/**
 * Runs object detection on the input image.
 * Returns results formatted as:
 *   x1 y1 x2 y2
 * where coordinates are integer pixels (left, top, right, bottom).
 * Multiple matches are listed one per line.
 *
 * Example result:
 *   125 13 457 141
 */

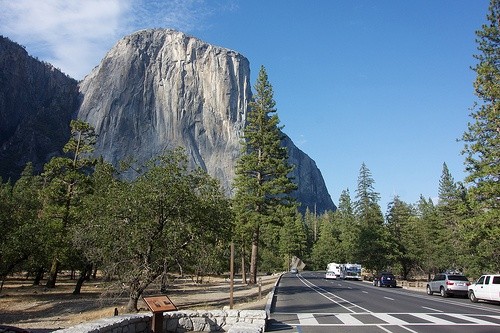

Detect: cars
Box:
323 271 337 280
372 272 397 288
290 267 298 274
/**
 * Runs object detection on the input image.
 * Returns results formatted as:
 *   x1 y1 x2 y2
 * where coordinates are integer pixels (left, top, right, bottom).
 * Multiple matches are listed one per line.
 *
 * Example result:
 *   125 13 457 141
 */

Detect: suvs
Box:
468 275 500 304
425 273 471 297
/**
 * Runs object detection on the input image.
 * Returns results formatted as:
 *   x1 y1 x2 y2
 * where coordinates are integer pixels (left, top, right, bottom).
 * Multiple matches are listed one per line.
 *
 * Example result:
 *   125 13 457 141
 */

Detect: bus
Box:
338 264 362 280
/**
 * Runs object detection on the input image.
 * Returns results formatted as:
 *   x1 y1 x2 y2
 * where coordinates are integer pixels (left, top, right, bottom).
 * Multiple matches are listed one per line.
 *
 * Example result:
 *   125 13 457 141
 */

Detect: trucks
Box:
326 262 340 276
